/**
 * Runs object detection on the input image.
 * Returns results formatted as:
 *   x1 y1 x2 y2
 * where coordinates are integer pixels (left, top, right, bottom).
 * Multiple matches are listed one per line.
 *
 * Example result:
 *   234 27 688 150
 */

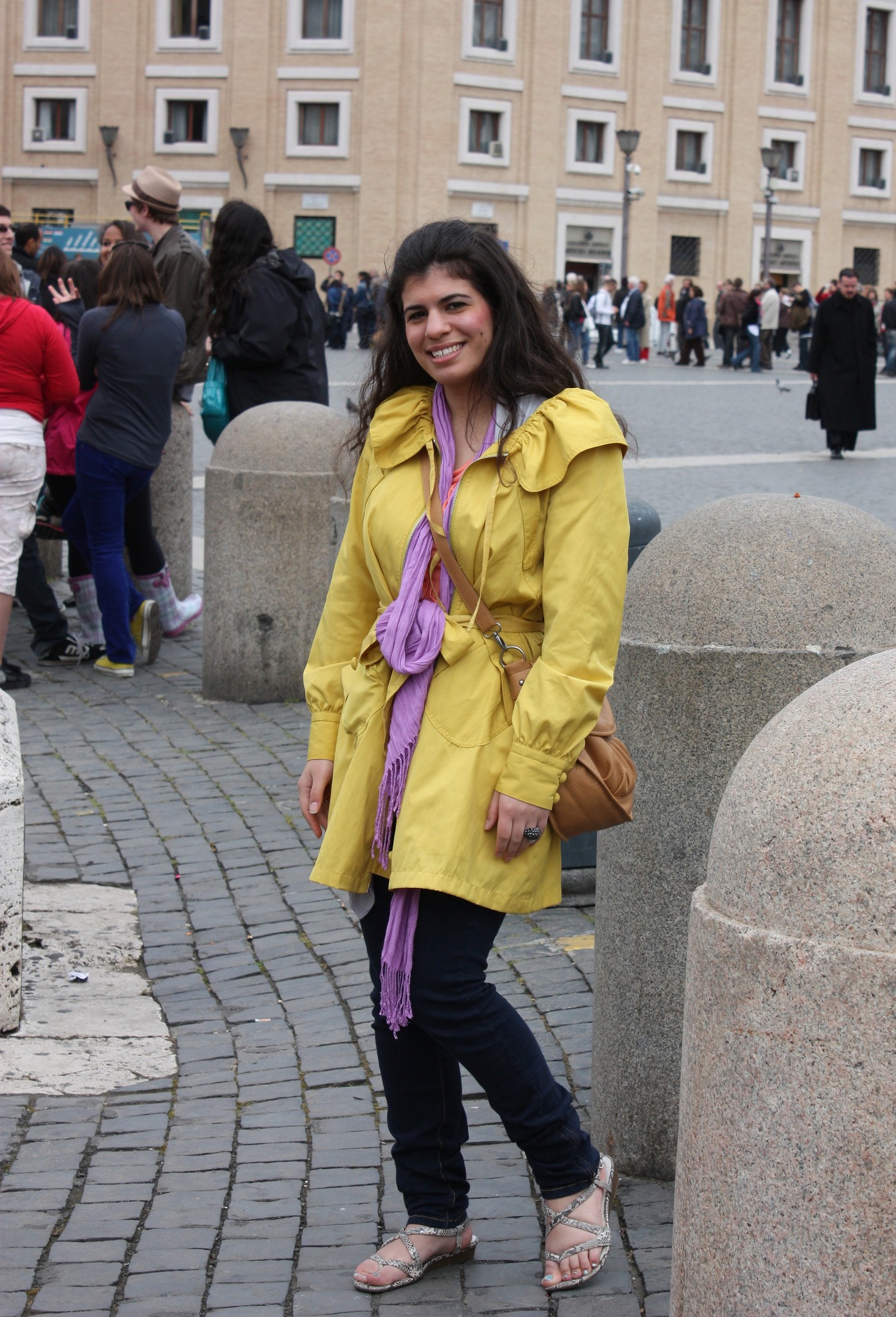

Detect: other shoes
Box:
830 449 844 459
658 350 704 366
613 347 647 365
594 357 603 368
785 349 792 360
62 597 75 607
581 363 594 368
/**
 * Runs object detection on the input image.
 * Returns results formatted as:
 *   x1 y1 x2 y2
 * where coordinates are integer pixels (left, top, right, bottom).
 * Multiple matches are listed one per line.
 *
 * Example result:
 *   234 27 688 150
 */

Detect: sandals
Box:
355 1213 478 1291
542 1151 615 1290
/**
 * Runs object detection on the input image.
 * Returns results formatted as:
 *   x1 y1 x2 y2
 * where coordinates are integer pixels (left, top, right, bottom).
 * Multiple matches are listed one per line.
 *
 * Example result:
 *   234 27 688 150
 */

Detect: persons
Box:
42 241 190 678
299 219 638 1293
0 163 212 664
317 265 896 381
198 200 330 448
0 242 80 689
804 268 877 460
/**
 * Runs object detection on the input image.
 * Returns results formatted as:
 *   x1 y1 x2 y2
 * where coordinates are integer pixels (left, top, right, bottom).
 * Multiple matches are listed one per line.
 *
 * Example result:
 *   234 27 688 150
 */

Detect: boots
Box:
66 573 107 656
135 562 204 636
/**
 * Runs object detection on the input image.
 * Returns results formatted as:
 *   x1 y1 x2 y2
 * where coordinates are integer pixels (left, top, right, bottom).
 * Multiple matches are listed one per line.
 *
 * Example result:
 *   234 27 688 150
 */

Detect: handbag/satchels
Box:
805 377 821 420
579 299 596 331
201 357 231 444
502 655 637 841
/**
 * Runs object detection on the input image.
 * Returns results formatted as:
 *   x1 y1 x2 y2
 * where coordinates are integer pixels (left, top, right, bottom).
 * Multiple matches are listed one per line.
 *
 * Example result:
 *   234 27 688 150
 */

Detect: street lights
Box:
759 146 785 281
614 129 642 278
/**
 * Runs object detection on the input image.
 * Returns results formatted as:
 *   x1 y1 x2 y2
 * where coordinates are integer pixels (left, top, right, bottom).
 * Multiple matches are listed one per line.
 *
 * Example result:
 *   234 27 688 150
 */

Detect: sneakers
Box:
94 655 134 677
130 599 160 663
37 632 94 662
0 655 32 690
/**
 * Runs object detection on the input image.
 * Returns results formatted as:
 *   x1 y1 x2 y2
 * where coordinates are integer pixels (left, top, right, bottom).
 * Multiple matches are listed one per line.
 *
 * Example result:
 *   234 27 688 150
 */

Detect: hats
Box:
122 166 183 213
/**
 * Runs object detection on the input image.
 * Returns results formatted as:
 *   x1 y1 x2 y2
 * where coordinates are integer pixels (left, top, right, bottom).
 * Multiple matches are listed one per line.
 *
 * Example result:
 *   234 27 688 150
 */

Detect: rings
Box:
525 827 541 840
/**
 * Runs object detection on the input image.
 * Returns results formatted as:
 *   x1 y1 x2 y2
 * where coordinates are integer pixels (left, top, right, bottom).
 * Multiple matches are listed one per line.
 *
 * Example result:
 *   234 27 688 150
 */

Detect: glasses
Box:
0 224 16 233
111 241 148 250
125 200 141 211
